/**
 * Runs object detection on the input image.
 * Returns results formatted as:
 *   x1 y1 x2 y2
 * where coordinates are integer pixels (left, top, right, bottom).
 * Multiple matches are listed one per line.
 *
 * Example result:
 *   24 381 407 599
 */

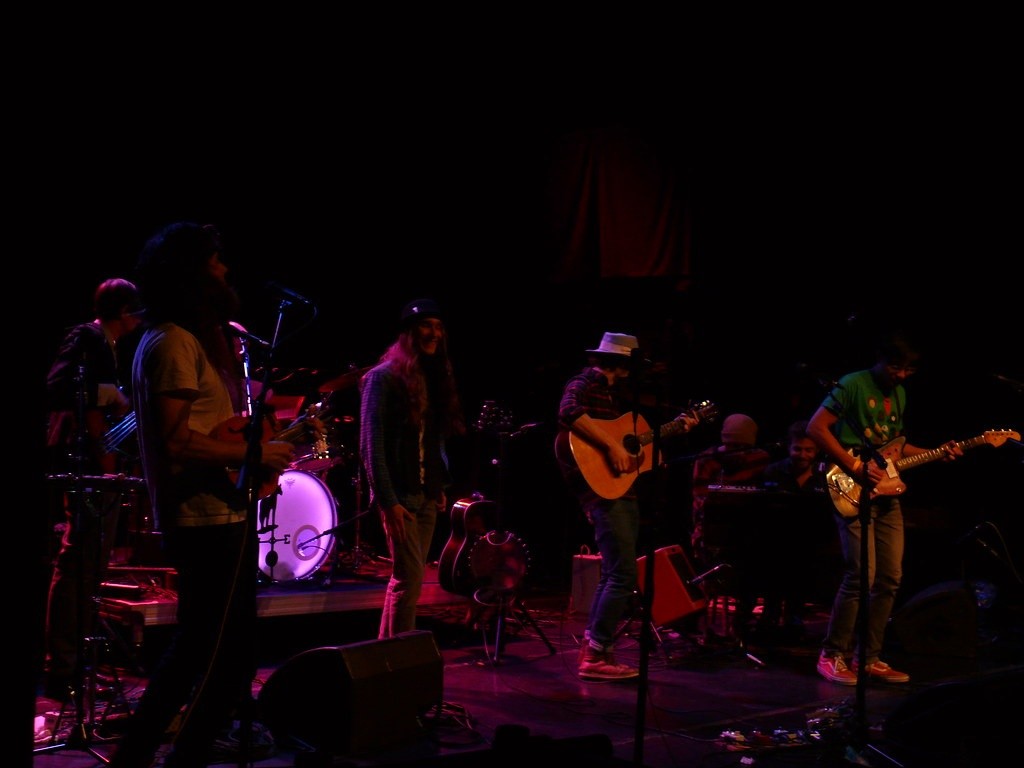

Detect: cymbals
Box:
317 366 374 393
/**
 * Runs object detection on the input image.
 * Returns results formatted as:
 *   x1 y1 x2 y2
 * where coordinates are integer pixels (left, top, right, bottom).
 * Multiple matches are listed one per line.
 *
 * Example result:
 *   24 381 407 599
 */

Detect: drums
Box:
286 419 343 472
125 455 163 536
256 468 339 581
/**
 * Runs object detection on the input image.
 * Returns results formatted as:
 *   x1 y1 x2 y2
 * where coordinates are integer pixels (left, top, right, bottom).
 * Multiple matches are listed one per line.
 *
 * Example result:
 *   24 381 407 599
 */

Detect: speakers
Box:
881 580 976 673
572 554 603 613
251 630 444 757
634 544 710 624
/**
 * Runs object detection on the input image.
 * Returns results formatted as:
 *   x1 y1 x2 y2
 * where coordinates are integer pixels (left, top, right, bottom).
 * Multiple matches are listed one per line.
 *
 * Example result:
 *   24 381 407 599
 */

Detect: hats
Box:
720 413 756 446
587 332 639 356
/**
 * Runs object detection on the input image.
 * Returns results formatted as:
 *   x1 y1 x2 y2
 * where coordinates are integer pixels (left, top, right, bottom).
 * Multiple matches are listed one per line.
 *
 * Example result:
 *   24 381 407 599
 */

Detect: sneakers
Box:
848 659 908 683
578 658 639 680
818 652 856 685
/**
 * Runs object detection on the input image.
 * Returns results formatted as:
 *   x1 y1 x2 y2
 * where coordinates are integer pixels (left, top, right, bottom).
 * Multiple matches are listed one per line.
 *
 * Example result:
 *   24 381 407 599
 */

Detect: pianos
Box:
692 479 826 503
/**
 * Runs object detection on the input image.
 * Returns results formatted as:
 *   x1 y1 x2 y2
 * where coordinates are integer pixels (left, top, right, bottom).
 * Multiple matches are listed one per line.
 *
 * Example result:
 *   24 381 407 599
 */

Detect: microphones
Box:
760 443 780 452
274 285 312 305
632 348 664 371
955 524 983 546
688 563 727 587
798 361 845 388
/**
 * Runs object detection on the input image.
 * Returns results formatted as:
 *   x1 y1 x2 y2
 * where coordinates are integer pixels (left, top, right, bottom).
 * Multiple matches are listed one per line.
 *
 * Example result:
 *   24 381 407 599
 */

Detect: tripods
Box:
31 491 132 765
333 453 383 576
456 431 559 663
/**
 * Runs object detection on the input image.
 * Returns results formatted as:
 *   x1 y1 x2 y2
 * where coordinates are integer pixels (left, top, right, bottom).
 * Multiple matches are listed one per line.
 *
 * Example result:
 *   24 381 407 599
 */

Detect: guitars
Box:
435 399 499 594
204 406 335 505
823 426 1022 520
83 400 138 475
554 398 719 506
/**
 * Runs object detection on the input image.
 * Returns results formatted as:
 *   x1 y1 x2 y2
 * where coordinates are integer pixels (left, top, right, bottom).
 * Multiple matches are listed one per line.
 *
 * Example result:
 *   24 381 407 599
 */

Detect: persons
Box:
554 332 699 679
358 300 467 639
44 278 146 697
132 222 328 768
806 327 963 686
691 414 836 655
